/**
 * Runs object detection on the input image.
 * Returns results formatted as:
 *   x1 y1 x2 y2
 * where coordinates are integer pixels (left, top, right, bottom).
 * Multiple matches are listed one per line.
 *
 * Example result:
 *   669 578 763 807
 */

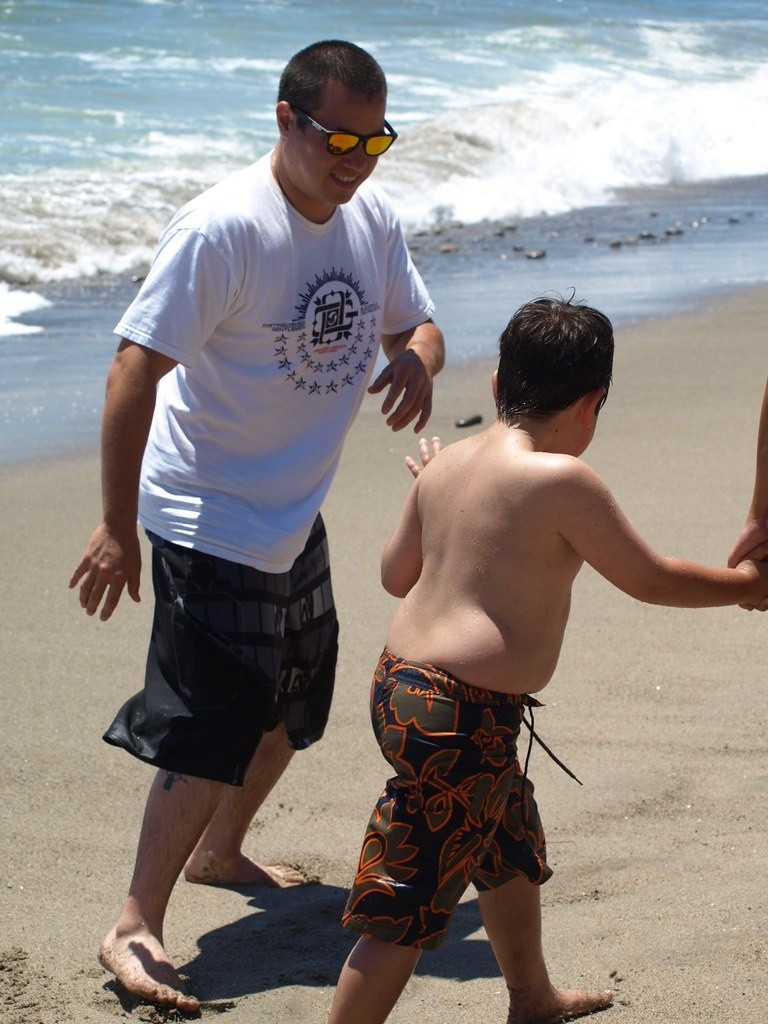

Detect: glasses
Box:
293 106 399 157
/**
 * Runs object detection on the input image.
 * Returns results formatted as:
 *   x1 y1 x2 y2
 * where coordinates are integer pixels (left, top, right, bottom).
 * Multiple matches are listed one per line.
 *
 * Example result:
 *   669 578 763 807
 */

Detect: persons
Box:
327 285 768 1024
70 40 445 1013
